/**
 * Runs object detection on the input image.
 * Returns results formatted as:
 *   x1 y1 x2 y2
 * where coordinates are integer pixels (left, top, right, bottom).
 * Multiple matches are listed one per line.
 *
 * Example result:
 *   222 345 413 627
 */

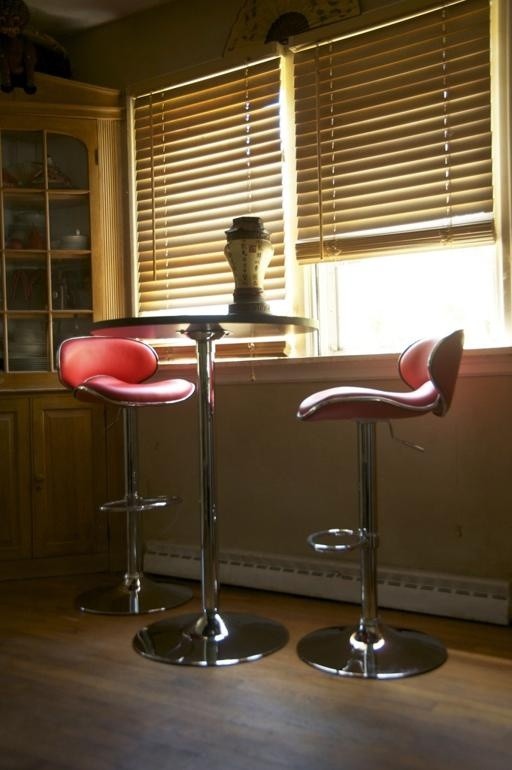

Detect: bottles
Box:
57 284 65 310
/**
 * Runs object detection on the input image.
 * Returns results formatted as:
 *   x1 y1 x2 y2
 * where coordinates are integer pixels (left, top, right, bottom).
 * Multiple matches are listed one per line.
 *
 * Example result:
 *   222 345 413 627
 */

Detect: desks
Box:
87 312 320 671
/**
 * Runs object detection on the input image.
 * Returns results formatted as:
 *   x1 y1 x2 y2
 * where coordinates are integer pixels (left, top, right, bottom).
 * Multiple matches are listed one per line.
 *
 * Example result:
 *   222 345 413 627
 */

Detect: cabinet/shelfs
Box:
1 388 126 582
1 68 129 389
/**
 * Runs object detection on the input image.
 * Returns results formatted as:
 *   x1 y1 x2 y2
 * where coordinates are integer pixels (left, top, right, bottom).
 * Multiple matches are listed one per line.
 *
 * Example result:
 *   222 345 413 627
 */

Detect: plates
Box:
0 355 50 372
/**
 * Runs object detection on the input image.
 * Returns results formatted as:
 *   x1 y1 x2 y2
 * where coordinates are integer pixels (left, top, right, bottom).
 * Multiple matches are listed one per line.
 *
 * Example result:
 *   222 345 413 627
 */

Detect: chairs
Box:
53 333 198 620
295 326 467 683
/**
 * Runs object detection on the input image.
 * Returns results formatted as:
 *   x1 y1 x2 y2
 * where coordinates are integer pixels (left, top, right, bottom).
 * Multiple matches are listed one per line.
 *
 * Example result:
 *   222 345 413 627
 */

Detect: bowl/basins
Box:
8 342 45 353
11 239 23 249
15 211 52 224
60 235 87 249
